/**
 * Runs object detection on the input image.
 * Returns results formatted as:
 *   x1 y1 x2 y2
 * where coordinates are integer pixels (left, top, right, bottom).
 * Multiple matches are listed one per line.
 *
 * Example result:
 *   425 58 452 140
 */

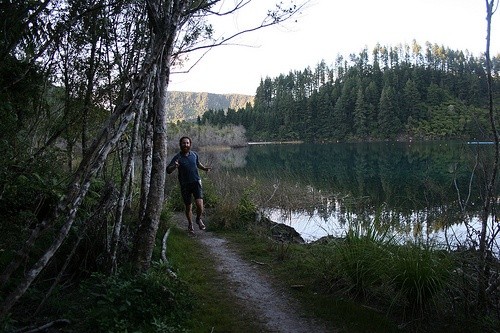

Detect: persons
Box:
166 137 212 234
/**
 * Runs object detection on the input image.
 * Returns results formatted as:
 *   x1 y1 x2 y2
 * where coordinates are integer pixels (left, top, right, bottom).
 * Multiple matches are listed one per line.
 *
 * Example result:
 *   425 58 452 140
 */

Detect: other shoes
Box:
188 222 194 233
196 217 205 230
